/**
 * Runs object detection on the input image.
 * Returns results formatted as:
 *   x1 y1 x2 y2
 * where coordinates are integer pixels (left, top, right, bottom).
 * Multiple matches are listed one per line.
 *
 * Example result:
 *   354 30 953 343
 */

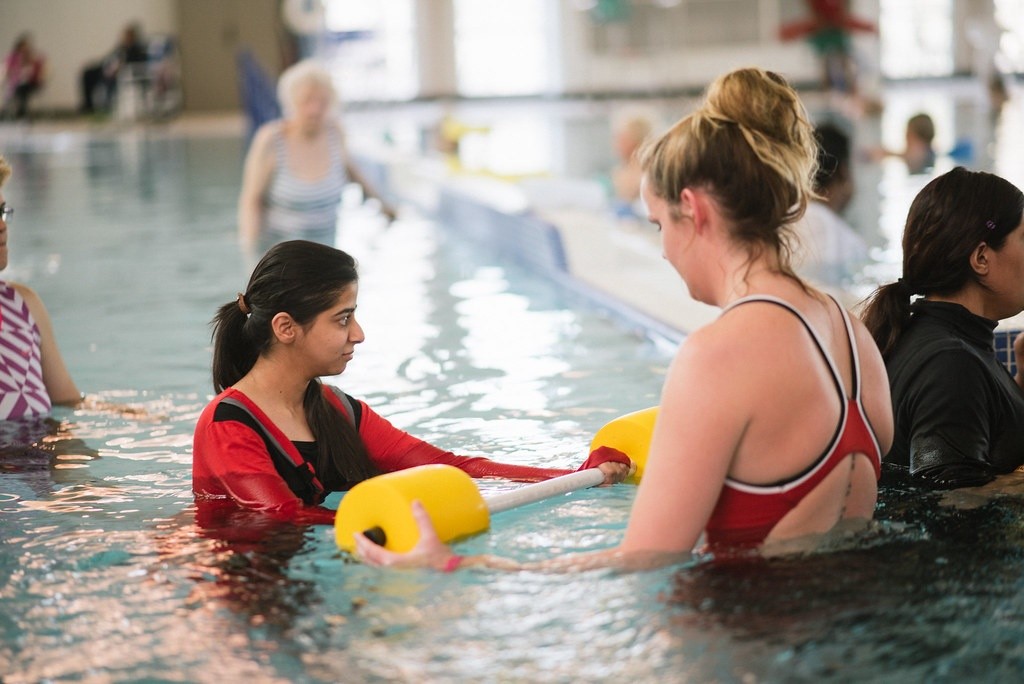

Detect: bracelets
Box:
444 557 463 571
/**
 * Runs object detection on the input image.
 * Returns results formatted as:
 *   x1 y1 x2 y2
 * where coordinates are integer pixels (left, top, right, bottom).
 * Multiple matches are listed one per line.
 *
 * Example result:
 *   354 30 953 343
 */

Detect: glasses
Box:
0 207 15 224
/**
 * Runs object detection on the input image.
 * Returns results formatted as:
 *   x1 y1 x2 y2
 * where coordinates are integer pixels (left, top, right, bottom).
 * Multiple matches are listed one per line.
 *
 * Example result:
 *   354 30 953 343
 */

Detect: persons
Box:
241 57 396 220
193 240 631 515
777 123 862 259
73 25 149 113
0 33 44 119
861 166 1024 473
0 158 81 422
907 114 935 149
353 69 894 565
